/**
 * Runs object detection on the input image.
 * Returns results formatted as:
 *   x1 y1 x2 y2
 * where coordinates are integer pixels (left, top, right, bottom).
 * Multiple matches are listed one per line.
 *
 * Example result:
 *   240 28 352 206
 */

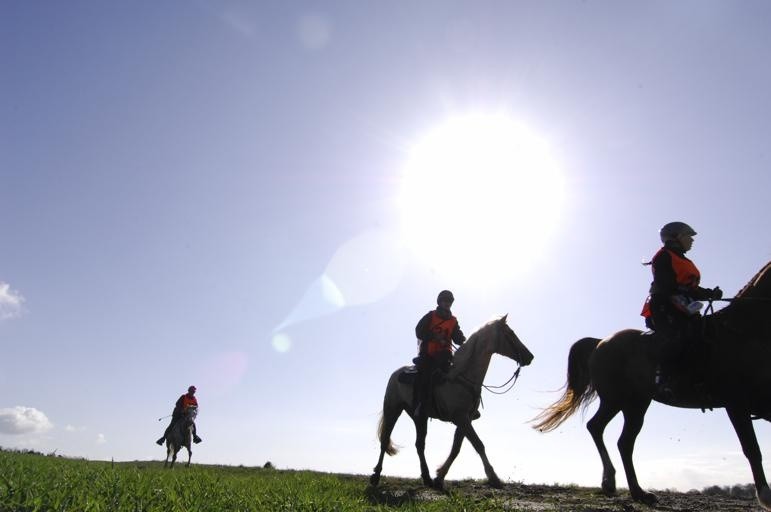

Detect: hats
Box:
189 386 196 392
437 290 454 304
660 221 696 241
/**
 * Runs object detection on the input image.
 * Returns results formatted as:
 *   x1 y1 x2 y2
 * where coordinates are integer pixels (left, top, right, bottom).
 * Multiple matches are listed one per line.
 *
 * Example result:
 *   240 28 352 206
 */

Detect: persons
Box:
156 385 202 445
413 287 482 420
640 220 725 405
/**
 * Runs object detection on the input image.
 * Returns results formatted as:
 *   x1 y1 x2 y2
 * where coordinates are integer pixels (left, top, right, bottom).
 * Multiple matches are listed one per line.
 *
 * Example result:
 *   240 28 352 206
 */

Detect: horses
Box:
164 403 199 470
533 261 770 512
368 310 533 492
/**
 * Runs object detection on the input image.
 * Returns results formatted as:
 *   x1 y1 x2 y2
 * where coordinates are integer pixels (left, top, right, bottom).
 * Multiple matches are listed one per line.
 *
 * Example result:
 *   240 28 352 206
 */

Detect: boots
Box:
193 436 200 443
158 437 165 444
